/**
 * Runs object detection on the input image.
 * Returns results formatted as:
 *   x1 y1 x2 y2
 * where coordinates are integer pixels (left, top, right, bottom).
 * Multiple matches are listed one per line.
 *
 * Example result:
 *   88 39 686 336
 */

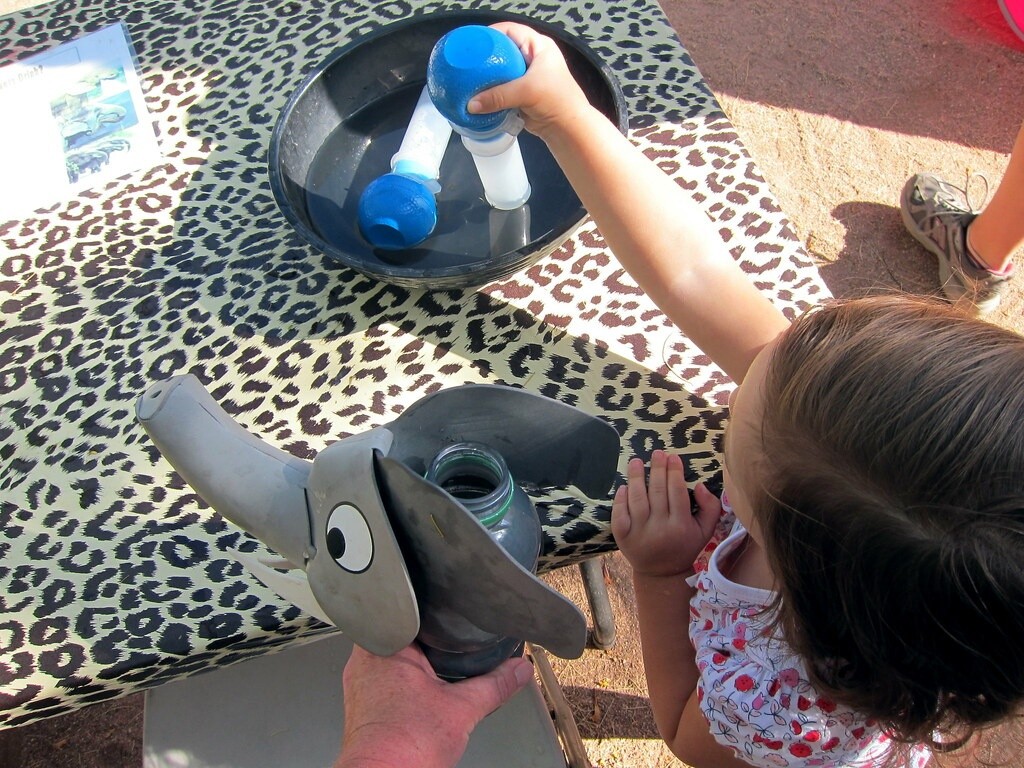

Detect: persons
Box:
900 118 1023 316
480 23 1024 767
331 639 533 768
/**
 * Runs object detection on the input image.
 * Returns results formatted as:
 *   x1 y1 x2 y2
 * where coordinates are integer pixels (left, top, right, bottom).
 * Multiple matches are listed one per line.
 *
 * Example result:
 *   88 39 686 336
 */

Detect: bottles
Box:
402 442 544 717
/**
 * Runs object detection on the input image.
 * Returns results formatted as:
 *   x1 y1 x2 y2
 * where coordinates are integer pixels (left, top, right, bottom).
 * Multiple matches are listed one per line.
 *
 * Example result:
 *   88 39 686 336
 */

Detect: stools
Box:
142 632 594 768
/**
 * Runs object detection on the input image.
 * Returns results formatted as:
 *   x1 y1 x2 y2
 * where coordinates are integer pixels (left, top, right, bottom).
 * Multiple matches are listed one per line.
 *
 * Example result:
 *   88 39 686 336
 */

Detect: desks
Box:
0 0 836 735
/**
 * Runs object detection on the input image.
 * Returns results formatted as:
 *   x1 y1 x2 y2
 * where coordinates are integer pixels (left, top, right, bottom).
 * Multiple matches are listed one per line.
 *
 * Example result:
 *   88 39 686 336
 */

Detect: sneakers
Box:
900 168 1015 316
